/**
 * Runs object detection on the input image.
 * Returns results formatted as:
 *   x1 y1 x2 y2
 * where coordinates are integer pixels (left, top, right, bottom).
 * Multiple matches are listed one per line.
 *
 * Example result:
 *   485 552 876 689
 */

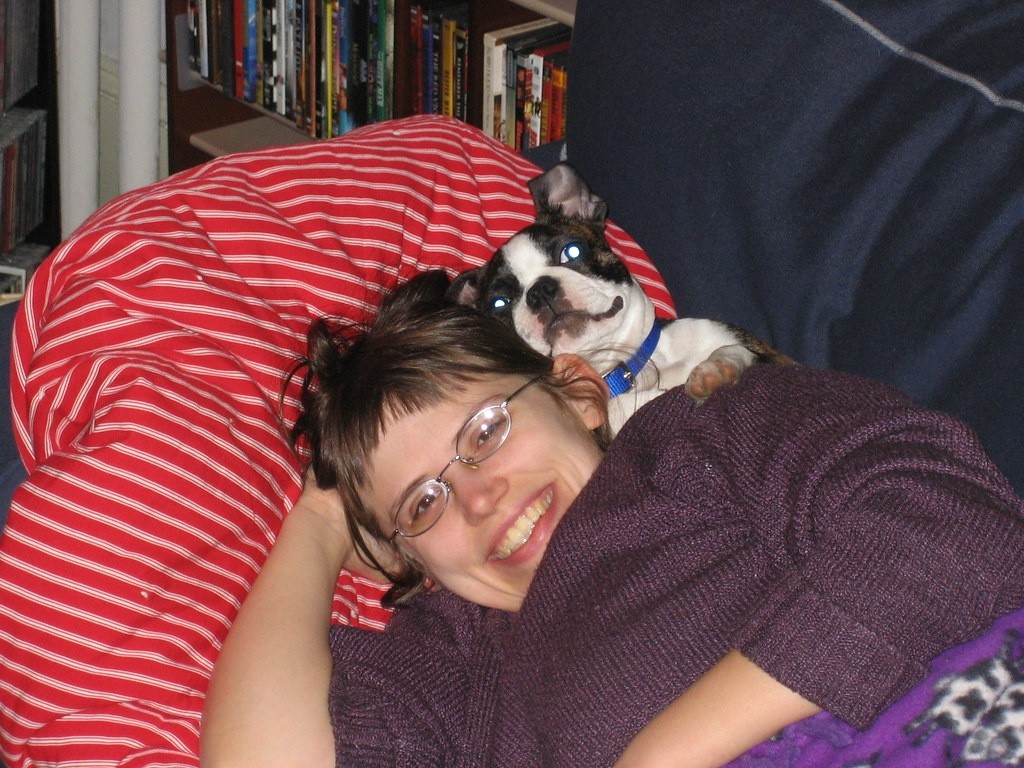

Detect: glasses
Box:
388 371 545 543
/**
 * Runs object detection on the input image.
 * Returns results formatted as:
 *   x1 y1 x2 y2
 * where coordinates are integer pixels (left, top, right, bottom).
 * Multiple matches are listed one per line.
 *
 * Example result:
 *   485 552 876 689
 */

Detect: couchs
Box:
0 0 1024 768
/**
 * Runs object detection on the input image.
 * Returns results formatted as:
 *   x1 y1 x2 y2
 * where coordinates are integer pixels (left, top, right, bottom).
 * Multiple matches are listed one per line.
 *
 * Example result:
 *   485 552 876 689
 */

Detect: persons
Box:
196 267 1024 768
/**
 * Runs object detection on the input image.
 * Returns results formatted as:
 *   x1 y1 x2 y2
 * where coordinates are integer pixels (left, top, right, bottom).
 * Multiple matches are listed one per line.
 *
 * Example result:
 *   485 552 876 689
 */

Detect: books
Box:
178 0 570 152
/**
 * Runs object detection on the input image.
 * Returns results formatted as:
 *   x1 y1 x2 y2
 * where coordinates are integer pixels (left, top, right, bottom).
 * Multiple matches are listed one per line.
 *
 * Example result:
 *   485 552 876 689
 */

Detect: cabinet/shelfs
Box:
169 0 577 172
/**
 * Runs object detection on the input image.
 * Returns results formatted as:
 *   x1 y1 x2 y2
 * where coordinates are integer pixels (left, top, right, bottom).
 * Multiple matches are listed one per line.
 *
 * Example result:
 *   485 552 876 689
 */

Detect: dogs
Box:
445 161 803 449
